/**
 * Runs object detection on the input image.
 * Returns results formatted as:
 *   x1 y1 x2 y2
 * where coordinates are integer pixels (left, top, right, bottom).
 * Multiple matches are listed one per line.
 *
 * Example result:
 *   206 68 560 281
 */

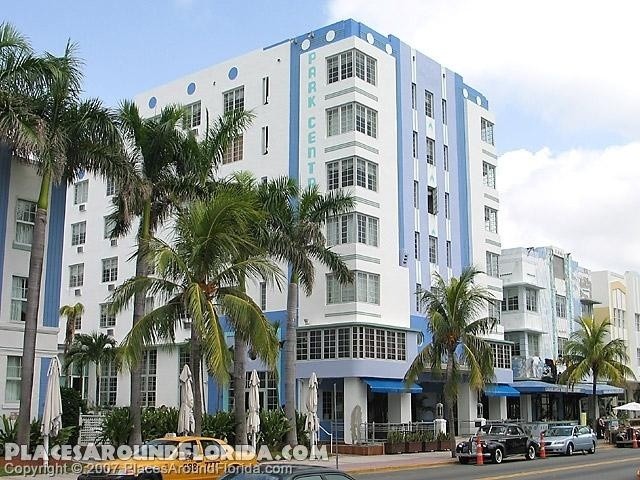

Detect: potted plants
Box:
384 428 451 454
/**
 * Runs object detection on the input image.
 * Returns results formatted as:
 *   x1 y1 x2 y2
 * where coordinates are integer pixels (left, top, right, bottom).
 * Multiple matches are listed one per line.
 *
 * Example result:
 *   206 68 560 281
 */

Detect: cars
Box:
616 418 640 448
455 423 541 464
77 433 357 480
538 425 597 456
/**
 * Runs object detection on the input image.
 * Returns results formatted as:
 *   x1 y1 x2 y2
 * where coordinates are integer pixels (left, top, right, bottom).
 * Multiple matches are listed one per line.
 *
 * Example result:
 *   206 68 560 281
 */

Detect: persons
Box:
599 416 607 435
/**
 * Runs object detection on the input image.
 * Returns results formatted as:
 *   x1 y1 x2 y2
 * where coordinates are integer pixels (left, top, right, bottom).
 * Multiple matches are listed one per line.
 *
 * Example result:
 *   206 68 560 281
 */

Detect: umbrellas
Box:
177 364 195 436
611 401 640 411
246 368 261 451
39 355 64 465
303 371 320 446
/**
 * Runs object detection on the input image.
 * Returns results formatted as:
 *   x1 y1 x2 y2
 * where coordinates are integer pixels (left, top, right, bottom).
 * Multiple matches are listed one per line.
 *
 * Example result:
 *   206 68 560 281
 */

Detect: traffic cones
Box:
632 430 638 448
476 436 483 465
540 433 545 459
